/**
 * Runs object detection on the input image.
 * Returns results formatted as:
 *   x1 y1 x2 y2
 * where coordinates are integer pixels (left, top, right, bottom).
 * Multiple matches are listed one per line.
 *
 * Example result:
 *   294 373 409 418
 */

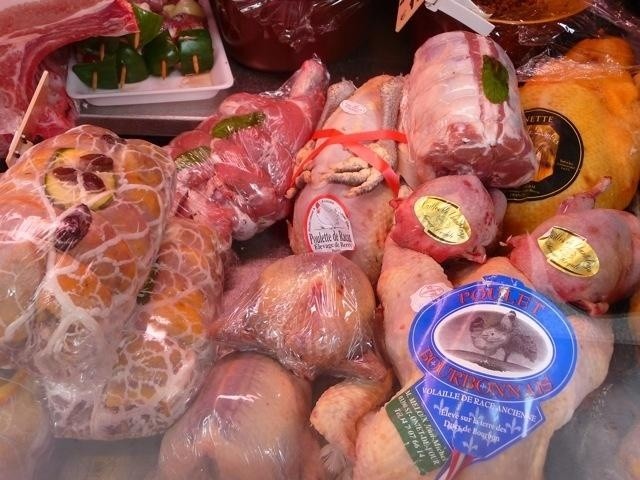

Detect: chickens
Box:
466 308 518 372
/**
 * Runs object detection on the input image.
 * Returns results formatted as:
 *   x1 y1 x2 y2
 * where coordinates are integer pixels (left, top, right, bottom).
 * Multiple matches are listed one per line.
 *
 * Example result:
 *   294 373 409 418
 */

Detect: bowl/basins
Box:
416 0 592 59
